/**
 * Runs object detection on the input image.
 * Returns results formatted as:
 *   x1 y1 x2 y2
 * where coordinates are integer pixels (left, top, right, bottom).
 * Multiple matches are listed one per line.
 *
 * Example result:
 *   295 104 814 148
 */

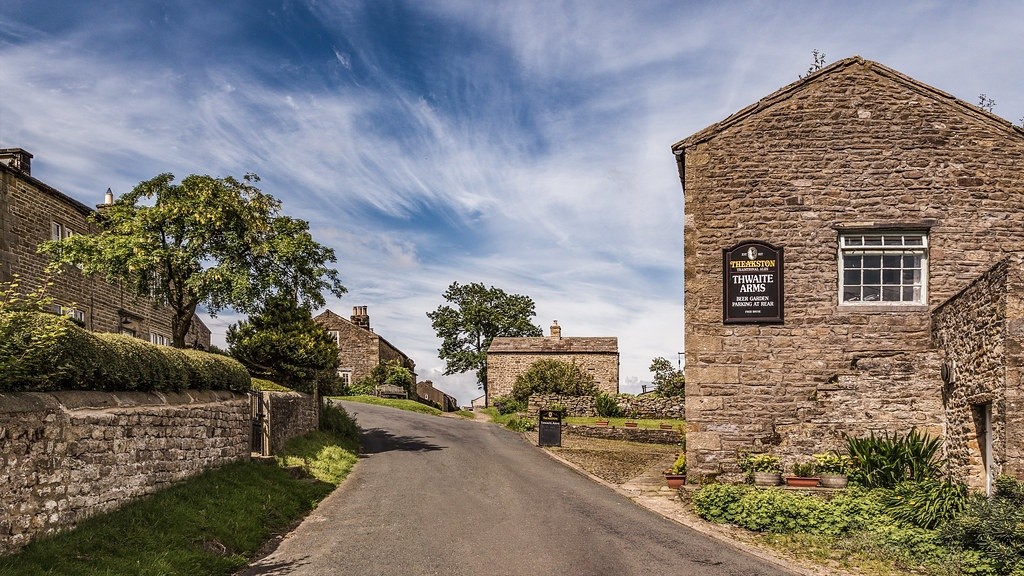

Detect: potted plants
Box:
665 453 686 489
747 453 785 486
812 451 860 488
624 418 638 427
786 461 821 487
659 420 673 429
595 415 609 426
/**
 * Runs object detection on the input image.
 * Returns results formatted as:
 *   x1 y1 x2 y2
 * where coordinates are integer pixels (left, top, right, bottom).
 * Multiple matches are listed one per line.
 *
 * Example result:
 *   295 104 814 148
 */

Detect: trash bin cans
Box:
538 407 562 447
